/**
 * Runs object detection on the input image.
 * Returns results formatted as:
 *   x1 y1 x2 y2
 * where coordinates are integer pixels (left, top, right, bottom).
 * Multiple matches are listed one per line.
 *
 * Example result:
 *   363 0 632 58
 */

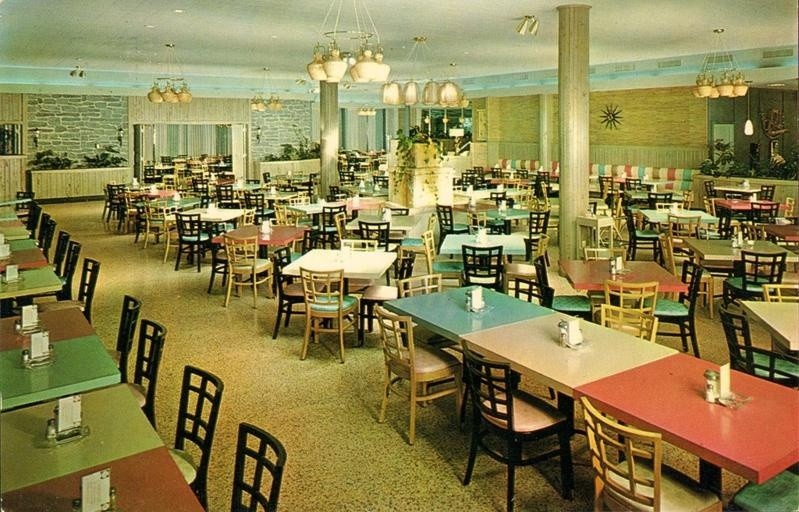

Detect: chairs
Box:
298 266 360 363
579 397 722 512
375 307 467 445
208 206 453 339
231 423 288 511
0 150 798 512
126 317 168 430
0 191 100 328
730 473 797 511
454 158 798 319
103 157 389 272
762 282 797 351
414 203 689 342
167 366 225 511
640 260 701 359
718 303 799 388
103 294 142 383
462 338 572 512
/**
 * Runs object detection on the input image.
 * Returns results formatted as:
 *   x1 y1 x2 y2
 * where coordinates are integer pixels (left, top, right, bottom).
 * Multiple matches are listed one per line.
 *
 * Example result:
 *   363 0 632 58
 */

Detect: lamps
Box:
70 65 86 78
33 127 40 148
116 126 125 147
423 109 466 124
256 127 262 143
356 102 377 116
252 66 283 111
381 36 471 109
512 16 541 38
742 79 754 136
148 41 195 106
692 26 748 101
307 0 392 84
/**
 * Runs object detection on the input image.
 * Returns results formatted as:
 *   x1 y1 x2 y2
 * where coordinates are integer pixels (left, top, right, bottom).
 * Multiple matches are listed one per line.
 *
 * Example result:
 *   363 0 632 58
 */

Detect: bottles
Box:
705 370 720 398
463 289 472 310
731 235 738 248
743 179 757 205
561 332 566 347
559 320 568 342
608 256 617 276
13 316 118 512
705 384 714 402
